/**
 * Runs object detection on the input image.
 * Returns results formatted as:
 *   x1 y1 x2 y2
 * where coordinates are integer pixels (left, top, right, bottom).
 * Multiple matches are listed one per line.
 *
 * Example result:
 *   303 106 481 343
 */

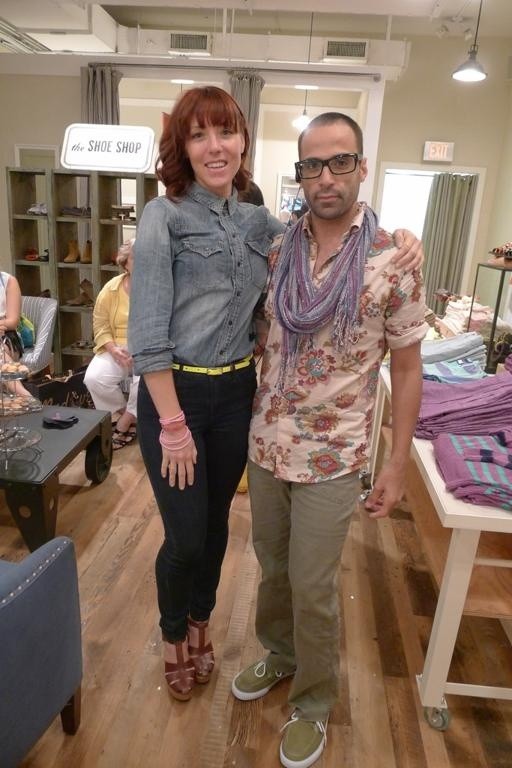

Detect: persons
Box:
126 85 426 701
231 112 425 767
83 238 141 450
0 272 37 401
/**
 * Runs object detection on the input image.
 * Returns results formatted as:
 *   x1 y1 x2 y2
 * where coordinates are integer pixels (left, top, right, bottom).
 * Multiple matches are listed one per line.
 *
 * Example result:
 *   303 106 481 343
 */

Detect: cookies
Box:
0 358 27 373
0 395 29 409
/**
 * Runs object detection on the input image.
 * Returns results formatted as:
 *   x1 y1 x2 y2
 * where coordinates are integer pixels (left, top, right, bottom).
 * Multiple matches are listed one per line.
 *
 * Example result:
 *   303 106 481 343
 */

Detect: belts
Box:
173 352 253 377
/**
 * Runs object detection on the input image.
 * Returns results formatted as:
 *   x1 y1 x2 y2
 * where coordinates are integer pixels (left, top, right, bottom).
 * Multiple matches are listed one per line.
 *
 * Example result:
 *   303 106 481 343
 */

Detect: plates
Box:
1 359 33 380
0 393 42 415
2 426 44 452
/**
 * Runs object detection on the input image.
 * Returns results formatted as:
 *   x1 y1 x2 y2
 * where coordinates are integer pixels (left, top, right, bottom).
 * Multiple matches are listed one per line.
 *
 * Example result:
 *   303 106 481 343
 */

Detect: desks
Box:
0 405 114 552
359 364 512 731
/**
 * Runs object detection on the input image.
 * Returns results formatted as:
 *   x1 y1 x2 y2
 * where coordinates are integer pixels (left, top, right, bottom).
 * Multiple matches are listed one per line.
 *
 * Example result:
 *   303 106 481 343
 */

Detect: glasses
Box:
294 153 360 179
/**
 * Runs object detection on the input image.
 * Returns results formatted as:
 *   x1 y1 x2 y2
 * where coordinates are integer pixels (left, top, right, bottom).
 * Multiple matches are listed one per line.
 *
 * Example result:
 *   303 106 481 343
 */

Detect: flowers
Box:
493 240 512 255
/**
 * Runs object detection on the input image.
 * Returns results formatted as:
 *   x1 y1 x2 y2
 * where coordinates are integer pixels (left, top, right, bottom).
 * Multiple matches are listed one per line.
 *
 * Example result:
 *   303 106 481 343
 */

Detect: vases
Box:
488 250 512 268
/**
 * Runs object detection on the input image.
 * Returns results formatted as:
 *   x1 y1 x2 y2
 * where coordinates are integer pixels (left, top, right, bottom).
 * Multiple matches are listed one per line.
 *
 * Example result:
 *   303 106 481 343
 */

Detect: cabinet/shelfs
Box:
273 174 304 220
6 166 165 377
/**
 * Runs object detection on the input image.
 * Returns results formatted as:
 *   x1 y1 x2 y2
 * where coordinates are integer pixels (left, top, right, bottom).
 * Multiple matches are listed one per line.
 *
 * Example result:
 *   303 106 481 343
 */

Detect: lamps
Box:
452 0 488 83
291 89 312 130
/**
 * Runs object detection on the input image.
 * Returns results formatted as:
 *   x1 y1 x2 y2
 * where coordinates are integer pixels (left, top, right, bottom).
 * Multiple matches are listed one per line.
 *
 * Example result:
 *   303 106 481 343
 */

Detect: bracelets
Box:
158 411 193 450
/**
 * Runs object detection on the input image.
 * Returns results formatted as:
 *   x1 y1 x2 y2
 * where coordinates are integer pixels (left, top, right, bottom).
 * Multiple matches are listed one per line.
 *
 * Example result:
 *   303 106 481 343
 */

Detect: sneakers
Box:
277 702 331 767
231 653 297 702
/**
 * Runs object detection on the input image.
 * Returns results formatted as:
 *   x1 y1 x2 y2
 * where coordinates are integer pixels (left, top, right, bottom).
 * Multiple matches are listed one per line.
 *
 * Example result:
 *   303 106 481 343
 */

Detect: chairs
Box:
1 536 83 768
18 296 58 375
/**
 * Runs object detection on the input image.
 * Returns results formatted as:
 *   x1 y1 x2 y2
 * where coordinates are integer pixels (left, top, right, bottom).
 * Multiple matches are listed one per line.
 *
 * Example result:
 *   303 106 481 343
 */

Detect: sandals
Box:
163 635 197 701
123 422 137 443
111 428 125 450
189 614 215 684
